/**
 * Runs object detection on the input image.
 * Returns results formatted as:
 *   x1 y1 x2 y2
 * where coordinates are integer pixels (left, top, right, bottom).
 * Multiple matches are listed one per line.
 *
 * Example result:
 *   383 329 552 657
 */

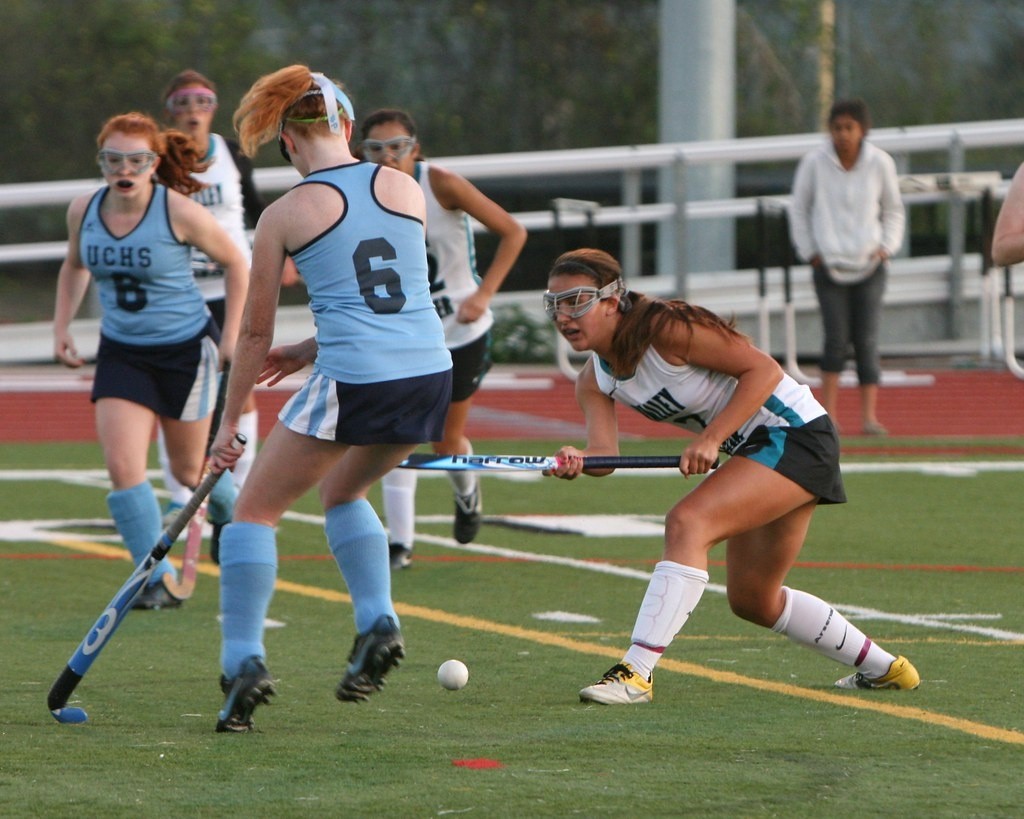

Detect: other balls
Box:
435 658 471 691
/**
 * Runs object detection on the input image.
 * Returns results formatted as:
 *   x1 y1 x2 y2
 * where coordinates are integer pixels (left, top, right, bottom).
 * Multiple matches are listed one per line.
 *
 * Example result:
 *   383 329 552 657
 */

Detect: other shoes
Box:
862 423 891 436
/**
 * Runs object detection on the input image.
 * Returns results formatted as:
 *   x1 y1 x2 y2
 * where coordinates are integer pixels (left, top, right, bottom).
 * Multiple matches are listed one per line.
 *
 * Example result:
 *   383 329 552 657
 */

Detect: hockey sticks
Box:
389 449 722 473
44 431 249 727
158 360 234 603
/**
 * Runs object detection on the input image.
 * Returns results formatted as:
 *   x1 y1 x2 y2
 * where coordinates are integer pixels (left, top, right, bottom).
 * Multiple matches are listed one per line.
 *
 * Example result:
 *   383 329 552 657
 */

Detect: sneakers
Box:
209 521 231 564
337 614 405 701
388 544 414 569
578 663 654 706
835 656 921 689
218 656 278 732
132 579 183 609
450 480 483 543
160 503 188 525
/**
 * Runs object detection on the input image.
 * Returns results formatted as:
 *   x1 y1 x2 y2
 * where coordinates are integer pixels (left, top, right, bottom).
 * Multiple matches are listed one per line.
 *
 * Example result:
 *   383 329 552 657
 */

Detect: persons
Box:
546 248 922 704
154 69 259 524
205 62 456 734
788 101 909 436
991 156 1024 266
358 111 528 572
51 114 250 606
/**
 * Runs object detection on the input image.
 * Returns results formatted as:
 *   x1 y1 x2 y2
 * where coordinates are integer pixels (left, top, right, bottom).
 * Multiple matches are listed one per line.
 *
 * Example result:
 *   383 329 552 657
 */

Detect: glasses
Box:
543 280 627 319
97 149 158 176
363 137 417 162
165 90 218 114
